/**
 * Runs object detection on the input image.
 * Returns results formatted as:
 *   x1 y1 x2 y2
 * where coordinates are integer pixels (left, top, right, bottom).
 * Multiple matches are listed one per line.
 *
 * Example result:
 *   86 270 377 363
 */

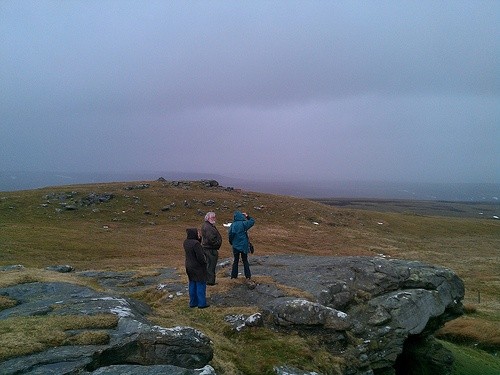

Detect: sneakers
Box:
190 305 198 308
198 305 210 308
209 283 218 286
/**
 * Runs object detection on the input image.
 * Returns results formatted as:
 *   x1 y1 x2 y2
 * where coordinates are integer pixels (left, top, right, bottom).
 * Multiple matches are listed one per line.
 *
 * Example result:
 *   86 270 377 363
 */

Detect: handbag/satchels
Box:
249 243 254 254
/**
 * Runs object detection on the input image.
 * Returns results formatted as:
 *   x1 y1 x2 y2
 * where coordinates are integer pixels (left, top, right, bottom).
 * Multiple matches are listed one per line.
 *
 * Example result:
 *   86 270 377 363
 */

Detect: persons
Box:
201 212 222 286
228 209 255 281
183 227 210 308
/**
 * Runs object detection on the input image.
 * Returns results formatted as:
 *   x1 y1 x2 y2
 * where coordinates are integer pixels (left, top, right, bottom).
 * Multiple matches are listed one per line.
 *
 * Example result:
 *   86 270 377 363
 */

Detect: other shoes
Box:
231 276 236 279
247 276 251 279
